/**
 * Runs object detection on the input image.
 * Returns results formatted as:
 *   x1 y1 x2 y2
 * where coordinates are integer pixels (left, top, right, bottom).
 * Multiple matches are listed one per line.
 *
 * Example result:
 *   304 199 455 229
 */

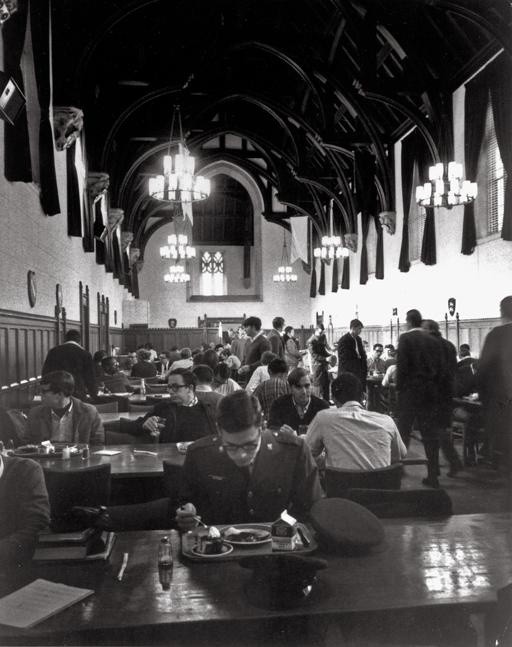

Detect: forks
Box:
181 505 221 539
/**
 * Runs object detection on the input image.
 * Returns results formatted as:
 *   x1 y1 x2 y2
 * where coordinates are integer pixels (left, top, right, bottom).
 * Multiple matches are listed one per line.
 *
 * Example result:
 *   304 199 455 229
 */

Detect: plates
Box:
219 524 272 545
176 441 194 454
192 541 234 557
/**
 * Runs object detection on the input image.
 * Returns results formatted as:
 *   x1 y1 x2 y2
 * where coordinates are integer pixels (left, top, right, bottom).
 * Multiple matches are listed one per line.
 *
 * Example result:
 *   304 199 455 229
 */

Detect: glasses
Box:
219 428 261 452
40 387 53 394
167 384 189 392
111 362 120 366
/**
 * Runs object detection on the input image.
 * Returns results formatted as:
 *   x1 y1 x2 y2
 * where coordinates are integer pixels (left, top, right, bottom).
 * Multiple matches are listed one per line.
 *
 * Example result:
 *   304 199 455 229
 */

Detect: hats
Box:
309 496 386 555
240 554 321 611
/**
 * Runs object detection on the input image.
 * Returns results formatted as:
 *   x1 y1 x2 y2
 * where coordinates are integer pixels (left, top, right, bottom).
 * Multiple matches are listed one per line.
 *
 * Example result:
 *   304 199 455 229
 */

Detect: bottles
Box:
140 378 146 400
159 535 174 571
81 443 90 460
159 568 172 591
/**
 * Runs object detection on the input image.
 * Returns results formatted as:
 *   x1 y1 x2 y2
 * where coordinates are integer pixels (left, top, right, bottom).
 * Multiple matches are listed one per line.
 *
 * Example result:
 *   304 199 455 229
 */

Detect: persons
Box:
172 390 324 531
22 296 512 444
307 372 408 471
422 319 465 478
0 441 52 578
395 310 443 489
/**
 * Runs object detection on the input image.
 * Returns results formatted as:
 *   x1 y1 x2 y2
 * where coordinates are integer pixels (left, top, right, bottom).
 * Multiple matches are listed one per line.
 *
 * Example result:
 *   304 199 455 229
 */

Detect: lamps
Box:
313 194 352 258
272 228 299 282
164 261 191 283
159 206 197 260
414 161 479 207
148 101 212 203
